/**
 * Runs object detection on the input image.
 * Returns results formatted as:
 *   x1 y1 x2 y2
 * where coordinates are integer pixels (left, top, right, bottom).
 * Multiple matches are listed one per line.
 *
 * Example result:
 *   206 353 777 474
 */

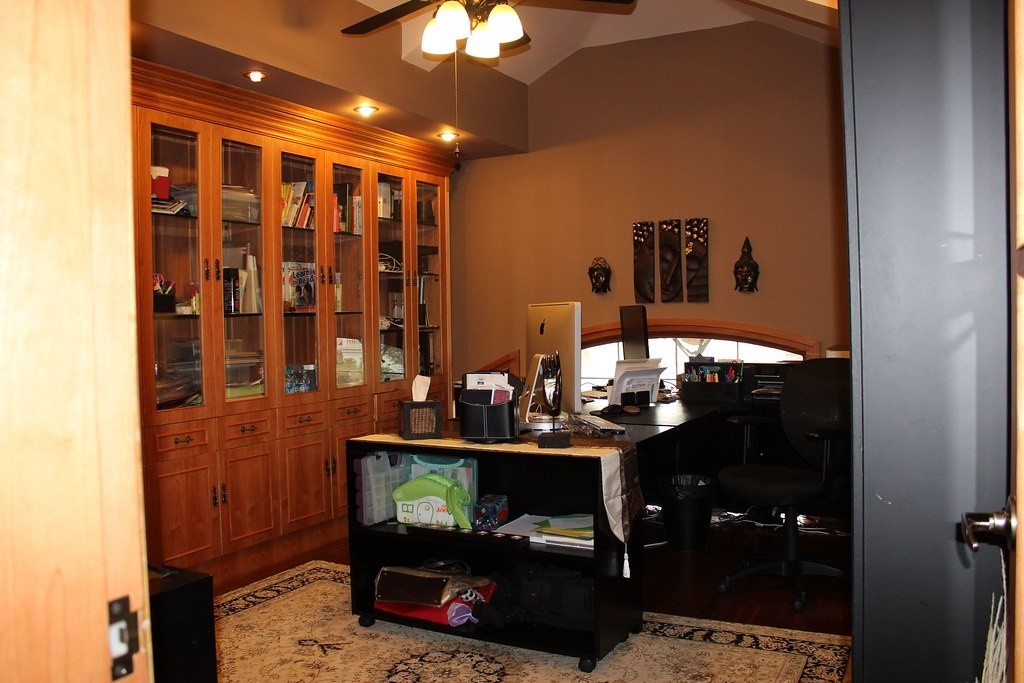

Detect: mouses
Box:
599 403 625 416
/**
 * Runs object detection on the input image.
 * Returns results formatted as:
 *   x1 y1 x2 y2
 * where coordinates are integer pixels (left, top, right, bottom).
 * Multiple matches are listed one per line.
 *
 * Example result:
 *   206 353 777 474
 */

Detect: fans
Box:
340 0 634 34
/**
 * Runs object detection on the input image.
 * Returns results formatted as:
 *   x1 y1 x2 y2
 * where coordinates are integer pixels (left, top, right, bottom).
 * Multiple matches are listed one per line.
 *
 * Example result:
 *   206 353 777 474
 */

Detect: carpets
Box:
211 560 851 683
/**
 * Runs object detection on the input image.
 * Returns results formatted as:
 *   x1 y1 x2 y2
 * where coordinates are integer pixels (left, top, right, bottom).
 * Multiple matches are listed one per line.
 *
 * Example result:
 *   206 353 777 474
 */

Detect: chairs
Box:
717 358 851 608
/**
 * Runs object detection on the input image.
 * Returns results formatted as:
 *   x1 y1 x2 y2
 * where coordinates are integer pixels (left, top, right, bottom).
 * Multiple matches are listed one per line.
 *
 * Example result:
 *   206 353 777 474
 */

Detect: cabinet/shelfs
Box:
347 432 642 672
129 55 455 595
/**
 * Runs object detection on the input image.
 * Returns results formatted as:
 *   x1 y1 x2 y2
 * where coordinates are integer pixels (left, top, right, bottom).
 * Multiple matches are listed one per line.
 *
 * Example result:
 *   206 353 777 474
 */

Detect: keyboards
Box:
570 415 626 438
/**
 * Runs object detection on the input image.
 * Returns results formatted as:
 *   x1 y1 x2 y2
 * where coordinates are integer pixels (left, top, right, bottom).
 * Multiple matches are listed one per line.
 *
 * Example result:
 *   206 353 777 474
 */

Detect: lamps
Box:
422 0 523 58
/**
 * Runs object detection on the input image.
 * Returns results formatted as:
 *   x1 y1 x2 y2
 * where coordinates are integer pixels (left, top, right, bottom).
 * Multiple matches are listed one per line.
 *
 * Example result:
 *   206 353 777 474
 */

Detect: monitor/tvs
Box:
514 297 583 427
616 303 650 360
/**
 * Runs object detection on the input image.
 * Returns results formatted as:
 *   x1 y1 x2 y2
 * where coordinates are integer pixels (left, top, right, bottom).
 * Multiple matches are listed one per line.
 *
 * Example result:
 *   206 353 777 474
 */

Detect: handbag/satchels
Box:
393 474 473 530
375 558 491 607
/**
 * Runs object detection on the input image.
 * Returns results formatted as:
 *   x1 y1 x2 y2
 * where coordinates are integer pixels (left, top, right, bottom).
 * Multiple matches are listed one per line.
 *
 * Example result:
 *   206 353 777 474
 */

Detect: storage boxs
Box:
683 361 746 404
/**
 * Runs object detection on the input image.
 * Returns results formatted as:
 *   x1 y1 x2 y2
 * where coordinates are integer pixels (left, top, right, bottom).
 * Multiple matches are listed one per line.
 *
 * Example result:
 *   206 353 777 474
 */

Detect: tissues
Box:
398 374 442 441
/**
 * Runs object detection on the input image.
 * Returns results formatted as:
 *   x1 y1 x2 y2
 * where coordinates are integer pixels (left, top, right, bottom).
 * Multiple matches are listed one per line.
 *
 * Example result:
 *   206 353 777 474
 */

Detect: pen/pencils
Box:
681 366 737 383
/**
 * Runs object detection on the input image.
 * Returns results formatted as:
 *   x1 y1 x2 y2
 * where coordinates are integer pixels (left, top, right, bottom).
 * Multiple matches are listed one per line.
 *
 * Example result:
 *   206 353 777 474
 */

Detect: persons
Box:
294 286 305 306
303 284 314 306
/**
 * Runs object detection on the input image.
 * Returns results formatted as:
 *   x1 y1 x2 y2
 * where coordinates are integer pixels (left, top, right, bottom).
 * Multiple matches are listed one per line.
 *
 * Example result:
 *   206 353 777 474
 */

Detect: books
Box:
282 261 342 313
753 371 784 401
281 179 315 230
332 182 363 236
530 514 594 550
378 182 403 221
223 243 263 314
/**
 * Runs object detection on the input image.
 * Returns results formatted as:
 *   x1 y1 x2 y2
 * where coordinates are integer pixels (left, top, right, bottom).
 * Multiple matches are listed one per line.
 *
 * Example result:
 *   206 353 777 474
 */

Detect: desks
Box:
598 422 674 447
606 399 719 477
